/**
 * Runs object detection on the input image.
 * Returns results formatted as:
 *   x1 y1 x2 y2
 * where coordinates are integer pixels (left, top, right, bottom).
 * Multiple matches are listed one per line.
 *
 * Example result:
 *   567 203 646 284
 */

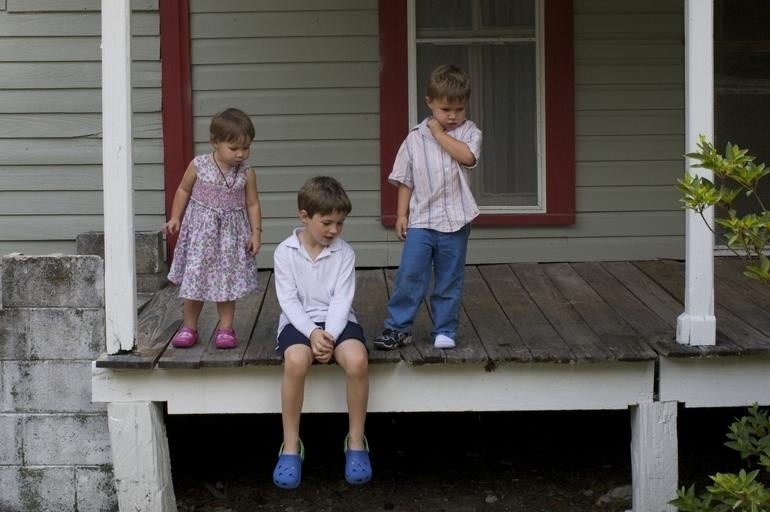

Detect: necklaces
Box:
212 151 239 194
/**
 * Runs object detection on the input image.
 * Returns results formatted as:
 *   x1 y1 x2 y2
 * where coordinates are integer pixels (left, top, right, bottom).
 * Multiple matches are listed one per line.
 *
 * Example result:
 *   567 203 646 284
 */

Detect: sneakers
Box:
372 327 413 352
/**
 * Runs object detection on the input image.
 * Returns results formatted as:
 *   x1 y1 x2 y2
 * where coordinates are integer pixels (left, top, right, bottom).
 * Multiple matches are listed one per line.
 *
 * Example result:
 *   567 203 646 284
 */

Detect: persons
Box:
162 106 262 348
272 175 374 492
373 65 483 348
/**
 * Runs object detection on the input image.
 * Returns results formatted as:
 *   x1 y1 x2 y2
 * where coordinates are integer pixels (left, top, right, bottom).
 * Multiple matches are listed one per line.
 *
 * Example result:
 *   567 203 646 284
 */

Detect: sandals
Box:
215 328 238 350
342 430 373 486
272 437 308 490
171 326 199 349
